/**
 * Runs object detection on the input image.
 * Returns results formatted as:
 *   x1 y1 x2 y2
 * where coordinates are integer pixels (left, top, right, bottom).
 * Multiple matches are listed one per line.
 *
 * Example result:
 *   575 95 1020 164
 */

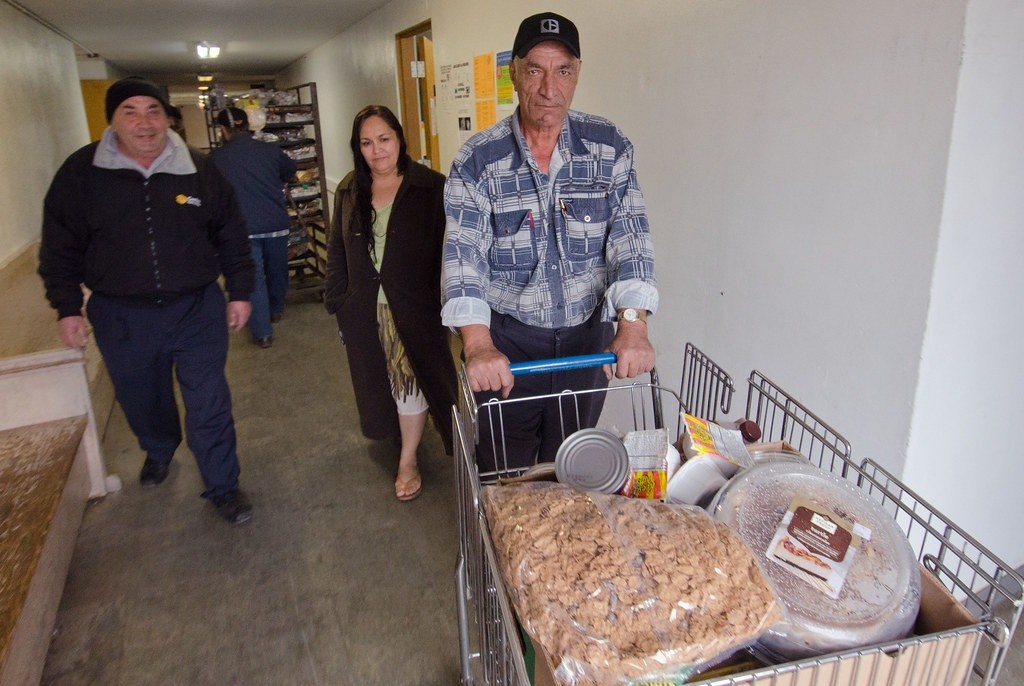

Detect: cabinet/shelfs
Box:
201 80 331 301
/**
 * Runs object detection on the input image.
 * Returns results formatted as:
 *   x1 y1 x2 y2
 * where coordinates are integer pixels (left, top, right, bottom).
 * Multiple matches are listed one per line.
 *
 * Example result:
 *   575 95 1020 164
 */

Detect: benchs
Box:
0 343 123 686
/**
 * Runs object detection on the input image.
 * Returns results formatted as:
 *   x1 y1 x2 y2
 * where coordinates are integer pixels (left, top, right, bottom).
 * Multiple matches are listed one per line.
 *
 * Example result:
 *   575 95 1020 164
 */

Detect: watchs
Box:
618 309 647 325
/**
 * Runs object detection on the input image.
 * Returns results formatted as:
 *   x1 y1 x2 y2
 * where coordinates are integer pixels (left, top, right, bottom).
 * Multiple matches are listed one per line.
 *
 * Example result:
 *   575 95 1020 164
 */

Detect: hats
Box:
216 107 248 128
510 11 580 61
105 75 165 123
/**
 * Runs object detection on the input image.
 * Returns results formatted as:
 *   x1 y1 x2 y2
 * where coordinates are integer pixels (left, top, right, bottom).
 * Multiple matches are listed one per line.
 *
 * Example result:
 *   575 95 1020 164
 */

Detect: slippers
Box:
394 472 422 501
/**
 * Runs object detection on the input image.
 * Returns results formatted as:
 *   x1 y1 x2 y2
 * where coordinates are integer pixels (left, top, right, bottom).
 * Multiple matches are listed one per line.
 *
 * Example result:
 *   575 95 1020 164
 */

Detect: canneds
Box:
554 428 629 495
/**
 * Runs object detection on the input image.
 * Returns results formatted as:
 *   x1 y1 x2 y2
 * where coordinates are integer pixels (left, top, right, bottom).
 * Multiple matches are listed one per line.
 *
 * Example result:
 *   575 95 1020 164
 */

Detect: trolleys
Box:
448 340 1024 685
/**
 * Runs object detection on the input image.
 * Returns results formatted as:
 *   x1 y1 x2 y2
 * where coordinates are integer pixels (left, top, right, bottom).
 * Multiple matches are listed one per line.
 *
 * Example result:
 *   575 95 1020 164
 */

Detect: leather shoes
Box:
252 336 273 348
138 457 172 490
270 314 282 324
212 489 254 528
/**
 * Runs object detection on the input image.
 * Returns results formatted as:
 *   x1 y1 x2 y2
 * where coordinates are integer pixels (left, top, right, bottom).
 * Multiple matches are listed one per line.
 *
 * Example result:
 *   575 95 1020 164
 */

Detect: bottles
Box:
739 420 761 444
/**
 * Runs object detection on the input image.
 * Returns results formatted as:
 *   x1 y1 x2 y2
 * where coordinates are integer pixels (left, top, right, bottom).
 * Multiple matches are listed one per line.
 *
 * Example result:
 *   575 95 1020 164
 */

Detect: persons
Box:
38 76 256 525
323 106 459 501
208 107 296 348
438 12 659 478
